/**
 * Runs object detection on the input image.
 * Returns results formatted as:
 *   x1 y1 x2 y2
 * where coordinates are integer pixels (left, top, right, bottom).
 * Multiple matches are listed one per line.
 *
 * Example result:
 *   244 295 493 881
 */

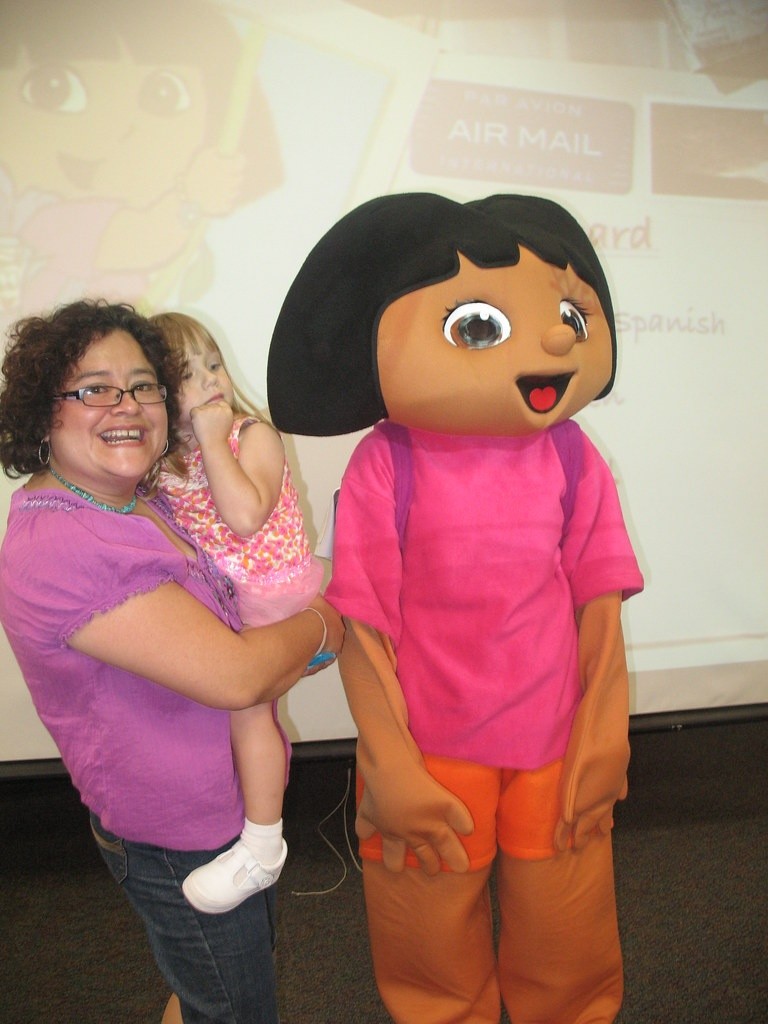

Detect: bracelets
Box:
300 606 336 675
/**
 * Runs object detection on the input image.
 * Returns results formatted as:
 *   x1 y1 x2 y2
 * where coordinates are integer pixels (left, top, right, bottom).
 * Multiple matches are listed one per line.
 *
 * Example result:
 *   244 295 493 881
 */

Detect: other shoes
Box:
182 836 288 914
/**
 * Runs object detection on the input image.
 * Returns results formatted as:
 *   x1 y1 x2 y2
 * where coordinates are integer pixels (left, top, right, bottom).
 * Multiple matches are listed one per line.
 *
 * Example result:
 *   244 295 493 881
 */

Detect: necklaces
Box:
47 463 139 514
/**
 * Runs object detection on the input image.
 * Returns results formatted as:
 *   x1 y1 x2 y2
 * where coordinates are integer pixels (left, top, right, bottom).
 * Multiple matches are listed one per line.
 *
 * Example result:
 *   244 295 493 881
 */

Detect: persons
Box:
146 314 324 916
0 301 344 1024
267 193 645 1024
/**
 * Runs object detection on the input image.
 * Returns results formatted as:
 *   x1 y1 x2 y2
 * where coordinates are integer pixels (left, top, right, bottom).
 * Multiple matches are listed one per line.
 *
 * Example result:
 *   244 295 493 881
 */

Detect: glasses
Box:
51 384 167 407
